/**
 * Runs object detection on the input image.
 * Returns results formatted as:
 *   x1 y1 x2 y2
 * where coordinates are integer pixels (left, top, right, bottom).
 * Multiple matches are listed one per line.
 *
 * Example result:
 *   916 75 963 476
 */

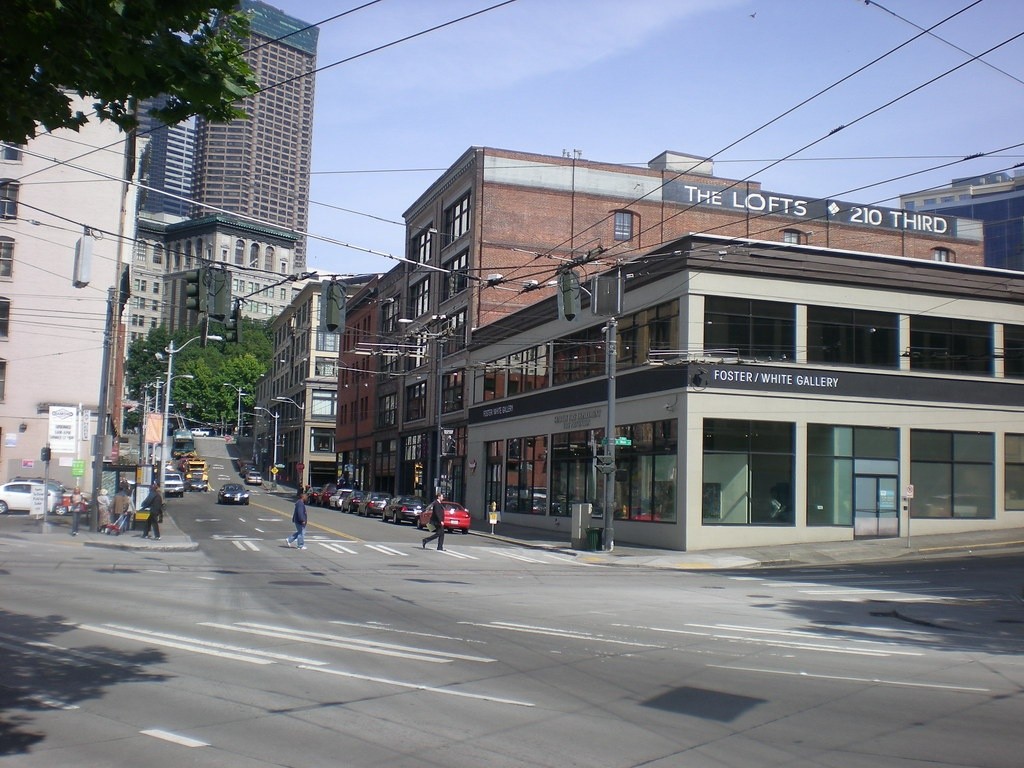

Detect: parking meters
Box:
39 443 52 534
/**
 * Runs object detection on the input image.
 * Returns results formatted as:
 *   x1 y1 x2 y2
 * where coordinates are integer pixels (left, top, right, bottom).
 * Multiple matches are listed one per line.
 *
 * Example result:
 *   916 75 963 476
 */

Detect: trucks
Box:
183 458 211 485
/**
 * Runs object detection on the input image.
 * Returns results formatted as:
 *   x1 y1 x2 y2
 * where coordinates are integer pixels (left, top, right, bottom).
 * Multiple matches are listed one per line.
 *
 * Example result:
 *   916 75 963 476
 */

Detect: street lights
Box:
398 317 443 499
277 396 305 498
254 407 280 491
548 281 616 552
223 383 246 435
143 335 222 503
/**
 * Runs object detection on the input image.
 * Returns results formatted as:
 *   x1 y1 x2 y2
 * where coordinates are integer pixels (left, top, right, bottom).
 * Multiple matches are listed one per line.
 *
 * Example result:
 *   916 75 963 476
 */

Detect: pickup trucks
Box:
189 428 209 437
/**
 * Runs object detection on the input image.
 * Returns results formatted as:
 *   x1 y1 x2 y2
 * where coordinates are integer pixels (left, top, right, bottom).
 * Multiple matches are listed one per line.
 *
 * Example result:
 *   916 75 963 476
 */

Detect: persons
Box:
109 487 136 531
286 493 308 549
296 483 311 503
139 484 164 540
97 489 110 534
70 486 85 536
423 493 446 551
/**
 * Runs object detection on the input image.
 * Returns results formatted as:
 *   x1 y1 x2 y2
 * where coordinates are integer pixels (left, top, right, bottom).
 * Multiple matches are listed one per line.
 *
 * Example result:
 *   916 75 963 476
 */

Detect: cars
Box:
217 484 250 505
417 500 471 535
164 474 184 499
301 487 423 524
178 458 188 471
184 478 208 492
0 482 80 515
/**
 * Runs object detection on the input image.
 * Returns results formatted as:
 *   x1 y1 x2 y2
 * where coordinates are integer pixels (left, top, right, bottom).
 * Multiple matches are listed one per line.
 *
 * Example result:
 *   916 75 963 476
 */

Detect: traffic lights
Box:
185 268 207 312
594 455 614 474
226 308 242 344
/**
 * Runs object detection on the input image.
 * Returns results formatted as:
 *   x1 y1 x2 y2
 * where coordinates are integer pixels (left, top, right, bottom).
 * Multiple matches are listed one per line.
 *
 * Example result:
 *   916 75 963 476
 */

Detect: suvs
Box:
245 471 262 486
237 457 257 478
9 477 92 513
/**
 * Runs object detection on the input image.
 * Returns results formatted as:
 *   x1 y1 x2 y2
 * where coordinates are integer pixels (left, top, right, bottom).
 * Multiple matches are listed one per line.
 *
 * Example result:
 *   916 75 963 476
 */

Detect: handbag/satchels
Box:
426 523 436 532
81 503 86 513
156 512 163 523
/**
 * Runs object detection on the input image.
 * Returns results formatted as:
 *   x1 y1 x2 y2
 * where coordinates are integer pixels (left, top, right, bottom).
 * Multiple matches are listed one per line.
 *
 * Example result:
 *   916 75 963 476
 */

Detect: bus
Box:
171 429 196 458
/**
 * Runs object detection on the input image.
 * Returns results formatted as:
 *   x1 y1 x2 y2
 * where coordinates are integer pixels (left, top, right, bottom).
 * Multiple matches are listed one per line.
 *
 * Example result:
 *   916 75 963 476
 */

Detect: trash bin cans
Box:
584 527 604 553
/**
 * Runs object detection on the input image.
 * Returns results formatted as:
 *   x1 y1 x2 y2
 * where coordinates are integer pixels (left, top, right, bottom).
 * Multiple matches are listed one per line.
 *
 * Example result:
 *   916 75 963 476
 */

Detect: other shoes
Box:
142 535 148 539
423 539 427 548
154 537 161 540
286 538 290 547
437 547 446 551
298 546 307 549
101 529 104 533
105 528 108 533
73 532 78 535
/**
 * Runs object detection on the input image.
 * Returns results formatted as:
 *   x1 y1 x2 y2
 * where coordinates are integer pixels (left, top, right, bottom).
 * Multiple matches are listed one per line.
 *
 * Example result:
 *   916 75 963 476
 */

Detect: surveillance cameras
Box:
666 404 669 408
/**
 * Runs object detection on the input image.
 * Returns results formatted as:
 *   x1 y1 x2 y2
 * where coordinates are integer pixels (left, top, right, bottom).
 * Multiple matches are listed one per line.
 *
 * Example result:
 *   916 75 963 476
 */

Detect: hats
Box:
99 489 107 495
